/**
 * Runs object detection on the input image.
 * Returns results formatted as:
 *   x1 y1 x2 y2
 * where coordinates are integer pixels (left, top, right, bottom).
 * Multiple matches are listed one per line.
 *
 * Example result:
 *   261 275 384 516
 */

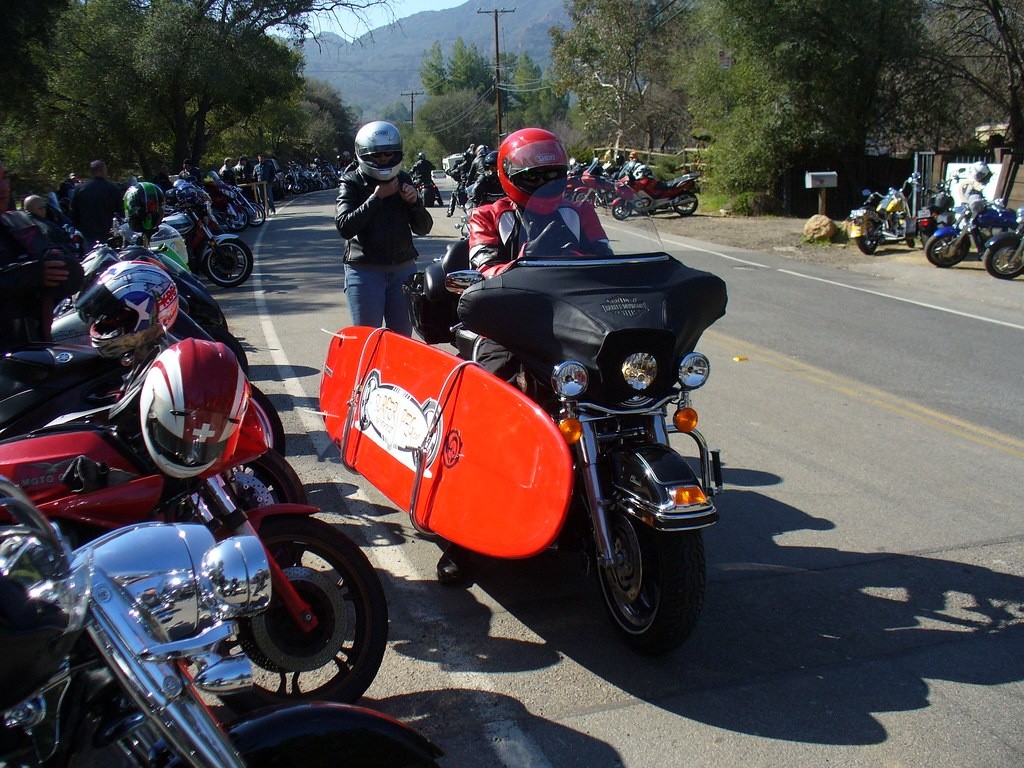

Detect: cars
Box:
247 159 285 199
431 170 446 178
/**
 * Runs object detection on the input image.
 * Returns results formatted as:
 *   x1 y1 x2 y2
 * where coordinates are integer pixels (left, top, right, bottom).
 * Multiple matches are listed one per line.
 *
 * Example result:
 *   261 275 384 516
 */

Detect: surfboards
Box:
317 325 574 559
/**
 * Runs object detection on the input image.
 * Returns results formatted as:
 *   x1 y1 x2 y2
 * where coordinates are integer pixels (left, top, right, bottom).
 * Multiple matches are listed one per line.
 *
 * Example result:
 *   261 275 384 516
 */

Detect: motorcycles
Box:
611 160 701 220
847 173 916 255
413 168 439 207
983 207 1024 280
924 182 1024 268
454 160 515 211
400 207 728 648
565 156 633 212
0 171 448 768
917 180 953 248
285 157 339 195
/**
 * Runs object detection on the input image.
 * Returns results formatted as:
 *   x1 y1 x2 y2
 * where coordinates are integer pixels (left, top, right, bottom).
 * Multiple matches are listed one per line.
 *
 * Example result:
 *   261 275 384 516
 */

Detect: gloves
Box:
561 249 591 256
517 243 527 257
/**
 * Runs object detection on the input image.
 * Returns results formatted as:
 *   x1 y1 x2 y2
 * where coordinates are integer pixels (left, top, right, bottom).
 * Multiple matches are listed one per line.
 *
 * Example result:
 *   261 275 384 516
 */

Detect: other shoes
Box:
447 213 453 217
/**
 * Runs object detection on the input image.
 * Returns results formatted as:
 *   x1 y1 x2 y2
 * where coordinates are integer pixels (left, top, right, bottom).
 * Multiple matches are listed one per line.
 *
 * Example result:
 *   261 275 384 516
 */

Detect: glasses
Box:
371 151 394 158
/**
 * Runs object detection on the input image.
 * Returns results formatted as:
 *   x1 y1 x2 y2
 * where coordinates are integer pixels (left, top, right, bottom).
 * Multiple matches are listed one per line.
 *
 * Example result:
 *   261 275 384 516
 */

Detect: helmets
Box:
476 144 489 158
140 337 250 477
176 183 195 201
484 151 498 166
355 121 403 180
971 161 993 184
89 260 179 357
498 128 569 214
124 184 164 232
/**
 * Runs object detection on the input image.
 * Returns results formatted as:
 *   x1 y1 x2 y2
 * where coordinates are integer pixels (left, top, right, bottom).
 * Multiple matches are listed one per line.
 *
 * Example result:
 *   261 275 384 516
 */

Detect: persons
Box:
437 127 615 589
465 151 506 210
408 151 443 206
341 150 354 167
24 194 72 229
0 163 70 353
153 166 174 192
218 157 237 186
253 154 276 218
178 159 203 186
445 143 490 217
56 172 75 200
72 160 123 244
334 121 433 338
628 151 643 164
234 155 253 183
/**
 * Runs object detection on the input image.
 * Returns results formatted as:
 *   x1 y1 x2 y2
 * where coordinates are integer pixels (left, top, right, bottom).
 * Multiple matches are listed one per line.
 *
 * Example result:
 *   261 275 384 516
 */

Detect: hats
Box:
224 157 232 163
418 152 426 158
182 159 191 164
